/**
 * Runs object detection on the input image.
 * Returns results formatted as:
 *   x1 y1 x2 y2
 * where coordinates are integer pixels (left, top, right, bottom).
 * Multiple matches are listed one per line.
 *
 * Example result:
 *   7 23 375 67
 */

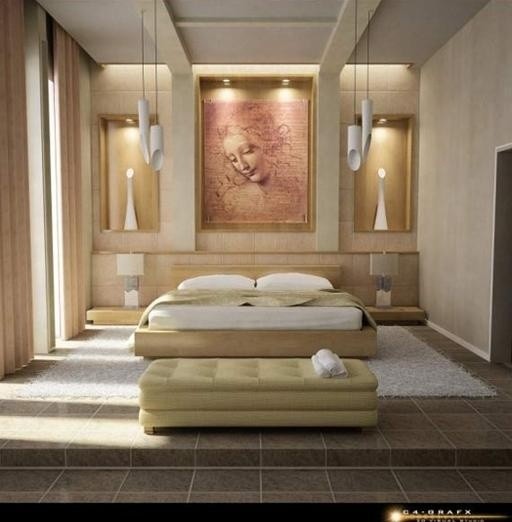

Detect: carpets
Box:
15 326 497 398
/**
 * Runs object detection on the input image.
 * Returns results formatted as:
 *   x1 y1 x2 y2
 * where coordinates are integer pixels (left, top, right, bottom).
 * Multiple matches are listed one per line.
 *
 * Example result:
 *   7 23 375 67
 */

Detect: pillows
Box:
177 274 255 290
256 272 334 290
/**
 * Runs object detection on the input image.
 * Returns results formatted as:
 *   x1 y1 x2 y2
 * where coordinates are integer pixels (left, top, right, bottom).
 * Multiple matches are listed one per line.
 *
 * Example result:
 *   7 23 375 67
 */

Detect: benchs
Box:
138 359 380 436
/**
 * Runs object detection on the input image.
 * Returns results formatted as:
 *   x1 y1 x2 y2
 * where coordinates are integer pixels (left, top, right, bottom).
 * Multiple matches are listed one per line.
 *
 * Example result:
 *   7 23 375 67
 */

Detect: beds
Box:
134 264 378 358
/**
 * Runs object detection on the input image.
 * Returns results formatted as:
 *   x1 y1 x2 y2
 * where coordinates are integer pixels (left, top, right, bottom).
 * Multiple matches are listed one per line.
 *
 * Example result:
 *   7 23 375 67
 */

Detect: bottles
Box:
385 278 390 290
378 277 383 289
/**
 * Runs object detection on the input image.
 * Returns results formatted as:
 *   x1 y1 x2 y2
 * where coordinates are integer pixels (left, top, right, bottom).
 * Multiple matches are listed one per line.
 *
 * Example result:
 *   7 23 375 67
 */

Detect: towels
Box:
310 349 330 379
315 349 340 374
331 352 349 378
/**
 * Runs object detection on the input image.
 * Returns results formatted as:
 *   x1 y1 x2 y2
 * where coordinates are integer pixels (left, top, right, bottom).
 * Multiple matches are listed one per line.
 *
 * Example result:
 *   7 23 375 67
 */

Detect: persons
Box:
216 102 303 223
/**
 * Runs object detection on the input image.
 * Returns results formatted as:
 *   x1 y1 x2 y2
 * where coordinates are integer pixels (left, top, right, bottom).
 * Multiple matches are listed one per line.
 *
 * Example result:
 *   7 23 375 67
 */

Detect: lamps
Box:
123 167 139 231
347 1 362 172
137 9 151 165
115 253 144 311
150 1 163 172
373 168 389 231
369 253 399 306
361 10 374 165
